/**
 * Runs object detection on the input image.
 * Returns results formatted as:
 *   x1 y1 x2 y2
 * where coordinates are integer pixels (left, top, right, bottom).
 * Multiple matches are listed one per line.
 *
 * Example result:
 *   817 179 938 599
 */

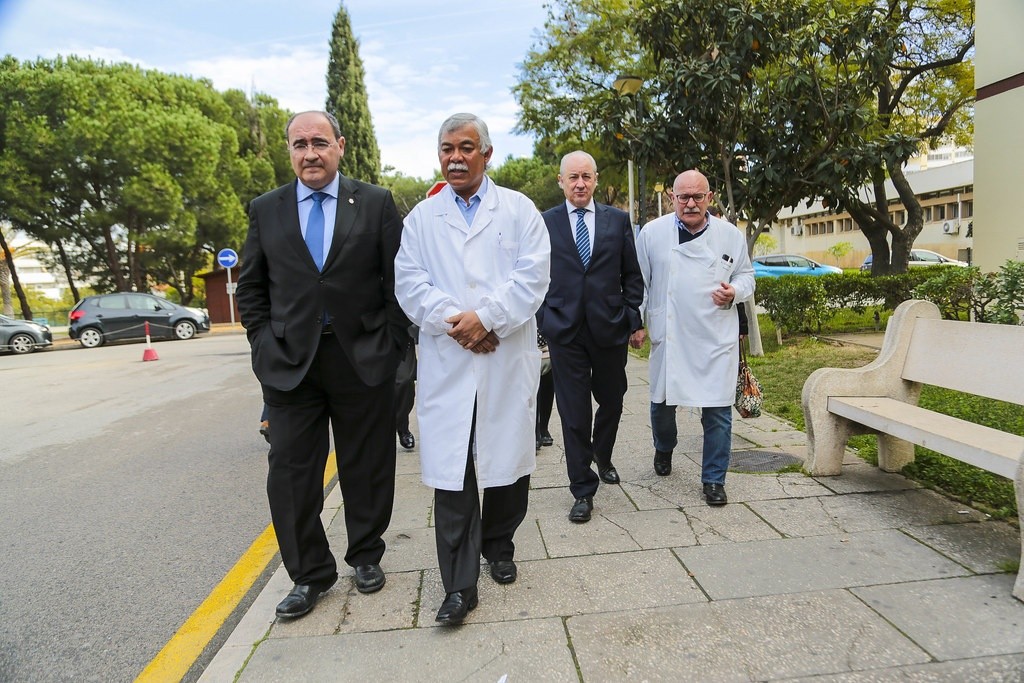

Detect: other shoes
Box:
260 421 270 444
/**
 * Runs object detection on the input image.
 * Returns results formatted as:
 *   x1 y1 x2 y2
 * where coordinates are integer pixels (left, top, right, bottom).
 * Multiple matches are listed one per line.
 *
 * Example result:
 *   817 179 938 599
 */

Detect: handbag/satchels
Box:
733 338 763 418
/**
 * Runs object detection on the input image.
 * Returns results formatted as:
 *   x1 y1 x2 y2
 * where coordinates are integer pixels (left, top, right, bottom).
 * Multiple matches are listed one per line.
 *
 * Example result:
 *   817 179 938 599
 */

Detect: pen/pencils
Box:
728 256 731 262
498 233 501 245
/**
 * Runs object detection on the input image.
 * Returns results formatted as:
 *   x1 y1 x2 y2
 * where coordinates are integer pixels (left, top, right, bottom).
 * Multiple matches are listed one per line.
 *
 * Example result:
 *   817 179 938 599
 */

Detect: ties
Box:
305 192 328 325
573 209 590 269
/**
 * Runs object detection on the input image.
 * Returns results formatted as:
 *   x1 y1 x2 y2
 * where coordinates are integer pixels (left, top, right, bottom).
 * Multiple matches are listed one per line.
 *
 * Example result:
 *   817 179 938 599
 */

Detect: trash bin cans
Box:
32 318 48 325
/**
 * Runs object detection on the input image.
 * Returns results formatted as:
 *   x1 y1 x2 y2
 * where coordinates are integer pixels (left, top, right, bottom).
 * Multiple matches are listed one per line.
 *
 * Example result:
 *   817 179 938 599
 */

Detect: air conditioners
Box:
943 220 958 234
791 227 801 236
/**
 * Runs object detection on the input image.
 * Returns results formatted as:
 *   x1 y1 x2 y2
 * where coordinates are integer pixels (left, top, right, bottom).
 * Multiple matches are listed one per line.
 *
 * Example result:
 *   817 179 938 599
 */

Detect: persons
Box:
234 109 419 622
393 111 554 624
535 149 645 522
628 170 756 508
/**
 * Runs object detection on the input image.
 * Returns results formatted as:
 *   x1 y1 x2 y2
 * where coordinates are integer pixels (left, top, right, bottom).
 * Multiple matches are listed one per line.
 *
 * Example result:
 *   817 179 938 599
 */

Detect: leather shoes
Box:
654 449 673 475
397 429 415 449
490 560 517 583
703 483 727 504
354 564 385 592
535 434 541 448
540 429 553 445
275 573 338 617
435 585 478 622
568 494 594 521
592 454 620 484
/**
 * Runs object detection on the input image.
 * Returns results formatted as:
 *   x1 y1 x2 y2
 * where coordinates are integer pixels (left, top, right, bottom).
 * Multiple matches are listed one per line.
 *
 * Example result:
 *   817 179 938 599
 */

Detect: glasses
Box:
674 194 707 204
289 140 338 154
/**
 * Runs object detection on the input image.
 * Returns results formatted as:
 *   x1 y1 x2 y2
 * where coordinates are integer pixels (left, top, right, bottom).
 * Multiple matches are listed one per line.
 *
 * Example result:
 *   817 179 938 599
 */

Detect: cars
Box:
752 255 843 278
859 249 969 275
0 315 53 355
68 292 211 349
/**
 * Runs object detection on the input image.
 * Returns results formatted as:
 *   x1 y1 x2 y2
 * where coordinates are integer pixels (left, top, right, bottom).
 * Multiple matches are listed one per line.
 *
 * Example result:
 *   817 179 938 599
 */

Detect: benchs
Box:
803 299 1024 602
